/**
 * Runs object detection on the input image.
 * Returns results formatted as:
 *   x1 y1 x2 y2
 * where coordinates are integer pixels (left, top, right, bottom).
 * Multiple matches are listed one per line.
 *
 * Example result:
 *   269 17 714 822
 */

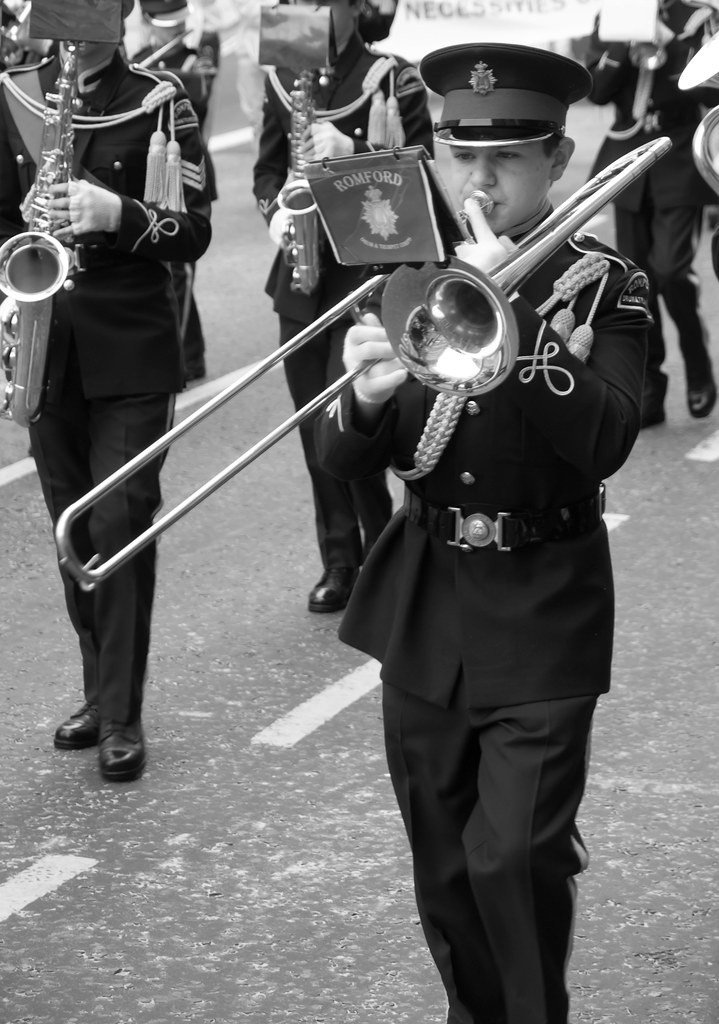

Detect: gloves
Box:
340 312 408 406
454 197 521 303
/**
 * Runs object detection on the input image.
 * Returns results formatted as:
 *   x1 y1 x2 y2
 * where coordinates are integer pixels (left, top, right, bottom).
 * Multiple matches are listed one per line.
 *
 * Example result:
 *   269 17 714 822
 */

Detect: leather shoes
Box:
641 406 665 428
687 360 717 418
53 703 101 749
308 566 360 612
99 718 146 781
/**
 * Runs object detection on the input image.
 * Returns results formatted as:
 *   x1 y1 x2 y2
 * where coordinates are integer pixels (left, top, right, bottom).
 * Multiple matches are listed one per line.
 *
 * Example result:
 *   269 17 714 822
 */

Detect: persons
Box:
249 0 437 611
315 41 655 1024
582 0 715 428
0 0 223 783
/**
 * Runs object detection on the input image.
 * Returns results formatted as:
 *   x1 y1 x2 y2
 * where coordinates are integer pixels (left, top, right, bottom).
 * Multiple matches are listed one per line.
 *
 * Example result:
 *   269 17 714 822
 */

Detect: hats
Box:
419 43 593 147
139 0 190 28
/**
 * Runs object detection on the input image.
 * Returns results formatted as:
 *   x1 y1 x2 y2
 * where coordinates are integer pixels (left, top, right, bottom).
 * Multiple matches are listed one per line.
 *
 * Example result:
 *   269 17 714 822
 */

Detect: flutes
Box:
137 27 196 69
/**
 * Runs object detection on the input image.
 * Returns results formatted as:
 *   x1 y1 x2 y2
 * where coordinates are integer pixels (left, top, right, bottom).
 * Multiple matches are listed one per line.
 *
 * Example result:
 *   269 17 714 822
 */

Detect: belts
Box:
644 105 674 132
402 484 606 552
65 239 143 271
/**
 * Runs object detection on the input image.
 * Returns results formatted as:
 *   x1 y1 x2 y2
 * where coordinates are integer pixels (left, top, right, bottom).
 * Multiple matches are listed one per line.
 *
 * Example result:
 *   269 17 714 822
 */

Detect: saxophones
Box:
277 69 321 296
0 42 81 428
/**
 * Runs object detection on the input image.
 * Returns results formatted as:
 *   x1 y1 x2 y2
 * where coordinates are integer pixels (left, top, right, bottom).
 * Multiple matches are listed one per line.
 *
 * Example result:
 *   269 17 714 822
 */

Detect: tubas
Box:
678 29 719 193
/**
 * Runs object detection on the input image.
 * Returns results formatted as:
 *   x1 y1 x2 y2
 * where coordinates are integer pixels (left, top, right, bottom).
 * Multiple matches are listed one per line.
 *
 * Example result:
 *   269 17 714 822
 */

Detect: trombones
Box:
629 37 667 118
55 133 673 587
